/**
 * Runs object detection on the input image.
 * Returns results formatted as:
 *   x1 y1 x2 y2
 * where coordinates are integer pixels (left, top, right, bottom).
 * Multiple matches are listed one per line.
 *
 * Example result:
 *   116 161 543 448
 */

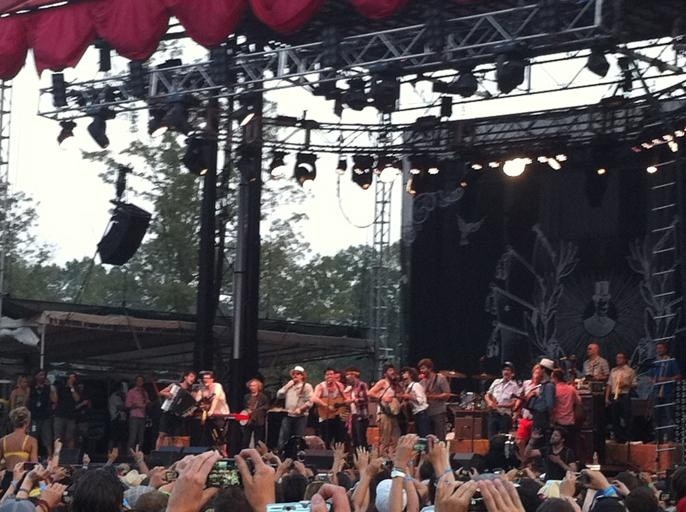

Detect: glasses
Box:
291 372 301 376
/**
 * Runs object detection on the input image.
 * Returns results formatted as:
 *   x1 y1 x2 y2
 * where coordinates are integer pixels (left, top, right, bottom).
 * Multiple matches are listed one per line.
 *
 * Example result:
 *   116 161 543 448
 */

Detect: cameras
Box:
575 473 588 483
413 438 427 450
266 500 331 512
24 463 38 470
166 471 179 483
517 471 527 477
660 492 673 501
207 458 253 490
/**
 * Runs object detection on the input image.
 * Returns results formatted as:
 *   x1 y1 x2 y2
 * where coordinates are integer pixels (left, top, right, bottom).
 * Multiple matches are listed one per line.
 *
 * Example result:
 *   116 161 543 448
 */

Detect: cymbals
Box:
440 370 467 379
473 374 494 381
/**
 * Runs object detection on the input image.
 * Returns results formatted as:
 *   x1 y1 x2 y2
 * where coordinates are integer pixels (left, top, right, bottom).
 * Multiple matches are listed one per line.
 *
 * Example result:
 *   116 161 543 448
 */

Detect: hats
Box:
536 358 556 373
501 361 516 372
289 366 308 379
376 480 408 512
119 470 146 488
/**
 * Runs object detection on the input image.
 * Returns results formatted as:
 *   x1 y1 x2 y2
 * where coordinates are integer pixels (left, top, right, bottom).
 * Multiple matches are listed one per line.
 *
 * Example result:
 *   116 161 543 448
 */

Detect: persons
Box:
583 298 615 336
1 342 686 512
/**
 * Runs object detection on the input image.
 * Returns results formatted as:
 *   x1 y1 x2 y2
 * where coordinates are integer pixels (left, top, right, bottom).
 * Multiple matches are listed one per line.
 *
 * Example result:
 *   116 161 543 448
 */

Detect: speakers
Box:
265 410 289 450
576 430 606 465
182 447 215 458
452 452 489 472
304 449 334 469
149 445 183 464
576 395 607 429
97 203 152 266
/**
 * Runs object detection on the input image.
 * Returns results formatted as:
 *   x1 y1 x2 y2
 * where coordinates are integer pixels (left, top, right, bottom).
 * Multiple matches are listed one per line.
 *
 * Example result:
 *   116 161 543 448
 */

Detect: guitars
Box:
510 393 534 406
313 396 352 421
512 384 542 410
